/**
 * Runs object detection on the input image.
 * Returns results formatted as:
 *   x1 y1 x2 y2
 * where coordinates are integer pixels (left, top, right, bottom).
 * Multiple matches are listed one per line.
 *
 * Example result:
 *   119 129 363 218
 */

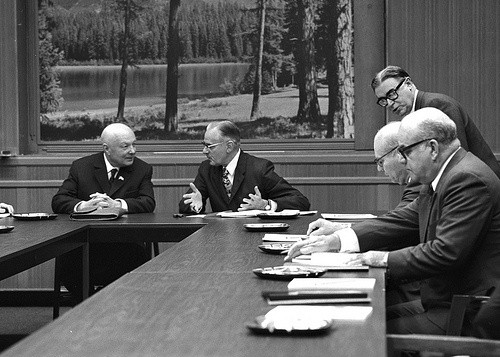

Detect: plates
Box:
258 242 297 254
12 213 58 220
243 223 290 233
246 313 333 333
252 265 329 280
0 225 14 233
257 211 300 219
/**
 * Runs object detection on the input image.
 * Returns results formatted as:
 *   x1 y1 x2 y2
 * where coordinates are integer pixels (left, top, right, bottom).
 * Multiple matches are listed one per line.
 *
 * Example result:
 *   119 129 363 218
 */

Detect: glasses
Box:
202 139 237 149
376 78 406 107
374 145 401 167
397 138 439 159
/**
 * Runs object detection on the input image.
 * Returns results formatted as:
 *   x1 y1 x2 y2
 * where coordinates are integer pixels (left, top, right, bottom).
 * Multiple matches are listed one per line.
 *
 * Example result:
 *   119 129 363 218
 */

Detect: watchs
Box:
265 201 270 210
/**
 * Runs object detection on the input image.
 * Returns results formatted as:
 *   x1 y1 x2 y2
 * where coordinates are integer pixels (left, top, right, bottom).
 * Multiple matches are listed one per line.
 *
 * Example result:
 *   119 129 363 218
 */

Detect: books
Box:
69 207 125 221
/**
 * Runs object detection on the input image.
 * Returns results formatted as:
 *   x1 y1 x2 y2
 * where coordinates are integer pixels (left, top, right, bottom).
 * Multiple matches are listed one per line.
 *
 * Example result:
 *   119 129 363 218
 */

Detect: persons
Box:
179 119 310 214
284 107 500 335
371 66 500 178
306 121 422 236
51 123 156 297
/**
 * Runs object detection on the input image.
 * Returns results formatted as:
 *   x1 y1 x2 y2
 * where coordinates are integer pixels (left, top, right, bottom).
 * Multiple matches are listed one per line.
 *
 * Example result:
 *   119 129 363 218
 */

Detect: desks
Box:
0 211 387 357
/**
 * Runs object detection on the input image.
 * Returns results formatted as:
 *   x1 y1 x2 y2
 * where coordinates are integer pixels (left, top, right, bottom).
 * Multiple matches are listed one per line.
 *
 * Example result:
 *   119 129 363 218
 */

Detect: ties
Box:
110 169 118 184
222 168 232 200
